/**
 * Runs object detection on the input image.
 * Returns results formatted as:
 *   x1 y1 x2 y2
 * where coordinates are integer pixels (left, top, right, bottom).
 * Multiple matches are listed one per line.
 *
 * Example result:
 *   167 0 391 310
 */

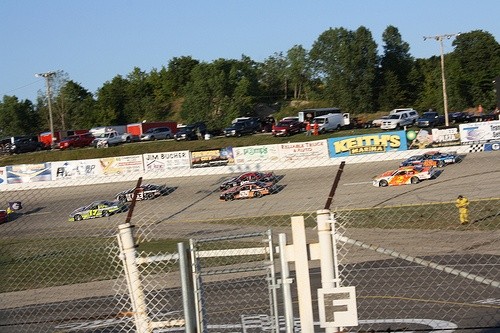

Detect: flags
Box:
8 201 23 211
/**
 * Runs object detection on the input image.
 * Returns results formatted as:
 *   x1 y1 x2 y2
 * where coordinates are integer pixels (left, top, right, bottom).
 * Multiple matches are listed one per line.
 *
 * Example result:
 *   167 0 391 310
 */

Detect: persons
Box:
456 194 470 226
204 131 213 140
477 103 484 117
313 121 319 136
306 121 312 137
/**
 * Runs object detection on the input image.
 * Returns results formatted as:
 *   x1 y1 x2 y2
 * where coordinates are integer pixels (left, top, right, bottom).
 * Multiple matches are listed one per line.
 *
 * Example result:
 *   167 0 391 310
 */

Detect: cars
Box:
220 172 273 190
68 200 128 221
371 118 383 127
220 180 278 201
448 111 498 124
0 210 14 225
400 150 458 167
140 127 174 141
372 166 436 187
8 137 44 154
113 184 168 202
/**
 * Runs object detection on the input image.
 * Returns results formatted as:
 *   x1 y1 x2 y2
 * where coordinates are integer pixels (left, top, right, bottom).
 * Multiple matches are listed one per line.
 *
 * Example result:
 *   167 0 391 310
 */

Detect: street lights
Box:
423 32 462 128
35 71 56 150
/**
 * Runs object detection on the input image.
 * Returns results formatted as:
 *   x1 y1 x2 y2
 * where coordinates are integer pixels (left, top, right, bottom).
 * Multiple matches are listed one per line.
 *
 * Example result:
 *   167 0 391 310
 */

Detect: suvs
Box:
57 133 96 151
272 120 300 137
223 120 258 138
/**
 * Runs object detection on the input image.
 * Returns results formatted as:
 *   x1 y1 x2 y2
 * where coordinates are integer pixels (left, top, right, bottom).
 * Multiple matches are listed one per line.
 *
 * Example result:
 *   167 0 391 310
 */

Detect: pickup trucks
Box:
381 110 418 131
92 129 132 148
415 112 445 128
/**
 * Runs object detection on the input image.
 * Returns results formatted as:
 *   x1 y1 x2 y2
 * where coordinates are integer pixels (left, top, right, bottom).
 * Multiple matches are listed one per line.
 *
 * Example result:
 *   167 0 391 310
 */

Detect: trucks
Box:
0 125 129 147
127 121 186 142
237 116 275 132
298 108 350 130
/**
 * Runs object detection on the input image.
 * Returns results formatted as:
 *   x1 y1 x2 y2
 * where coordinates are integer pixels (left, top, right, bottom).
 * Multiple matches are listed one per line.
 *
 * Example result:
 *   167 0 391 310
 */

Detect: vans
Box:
390 108 412 115
174 122 206 142
311 112 349 134
